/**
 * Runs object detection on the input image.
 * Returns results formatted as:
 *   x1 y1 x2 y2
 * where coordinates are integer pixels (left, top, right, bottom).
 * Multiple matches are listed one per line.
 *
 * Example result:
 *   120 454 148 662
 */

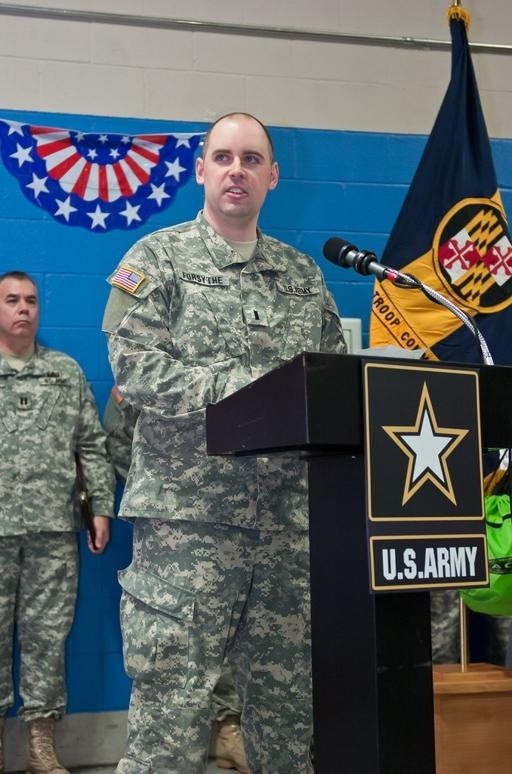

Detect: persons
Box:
104 107 352 774
431 586 512 680
1 271 118 771
102 374 253 771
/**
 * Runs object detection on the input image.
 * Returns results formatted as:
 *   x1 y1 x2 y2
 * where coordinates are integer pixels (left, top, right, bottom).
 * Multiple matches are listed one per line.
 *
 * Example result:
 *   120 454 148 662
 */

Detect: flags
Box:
368 0 511 499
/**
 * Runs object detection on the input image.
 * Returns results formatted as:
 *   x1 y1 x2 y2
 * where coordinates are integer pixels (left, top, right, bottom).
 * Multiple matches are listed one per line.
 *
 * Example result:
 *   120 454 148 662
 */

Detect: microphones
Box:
324 237 399 283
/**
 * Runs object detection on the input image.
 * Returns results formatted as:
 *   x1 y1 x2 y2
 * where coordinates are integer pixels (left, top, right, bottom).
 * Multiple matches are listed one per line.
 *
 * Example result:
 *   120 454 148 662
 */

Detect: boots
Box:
215 712 255 773
26 717 72 774
